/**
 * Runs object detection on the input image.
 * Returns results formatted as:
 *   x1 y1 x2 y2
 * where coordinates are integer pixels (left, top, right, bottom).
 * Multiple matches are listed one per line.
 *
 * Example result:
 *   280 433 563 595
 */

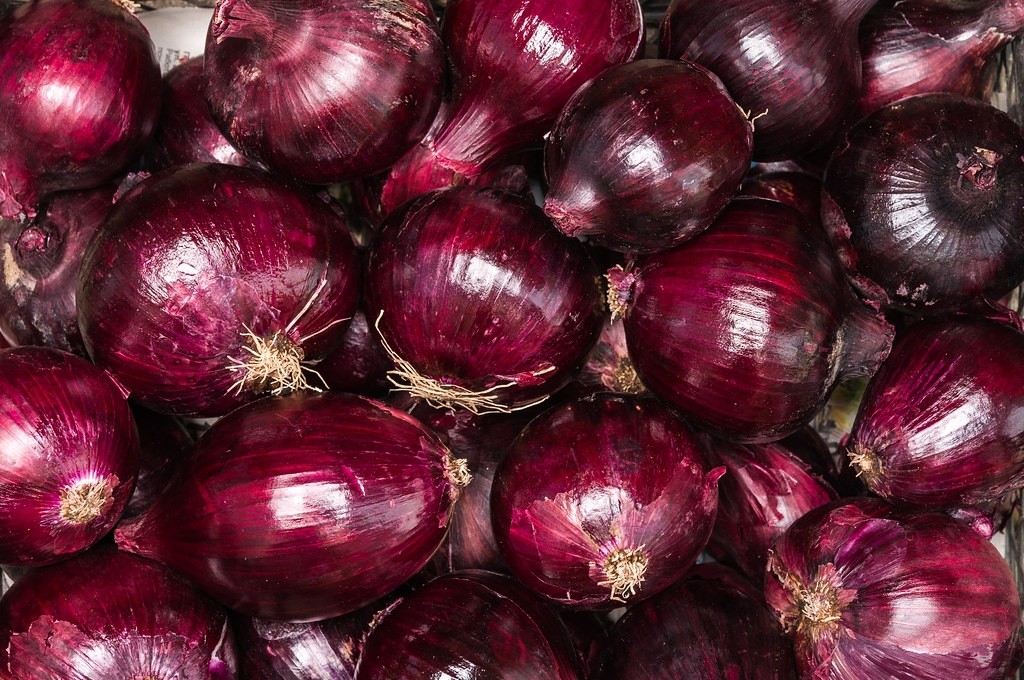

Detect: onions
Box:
0 0 1024 680
